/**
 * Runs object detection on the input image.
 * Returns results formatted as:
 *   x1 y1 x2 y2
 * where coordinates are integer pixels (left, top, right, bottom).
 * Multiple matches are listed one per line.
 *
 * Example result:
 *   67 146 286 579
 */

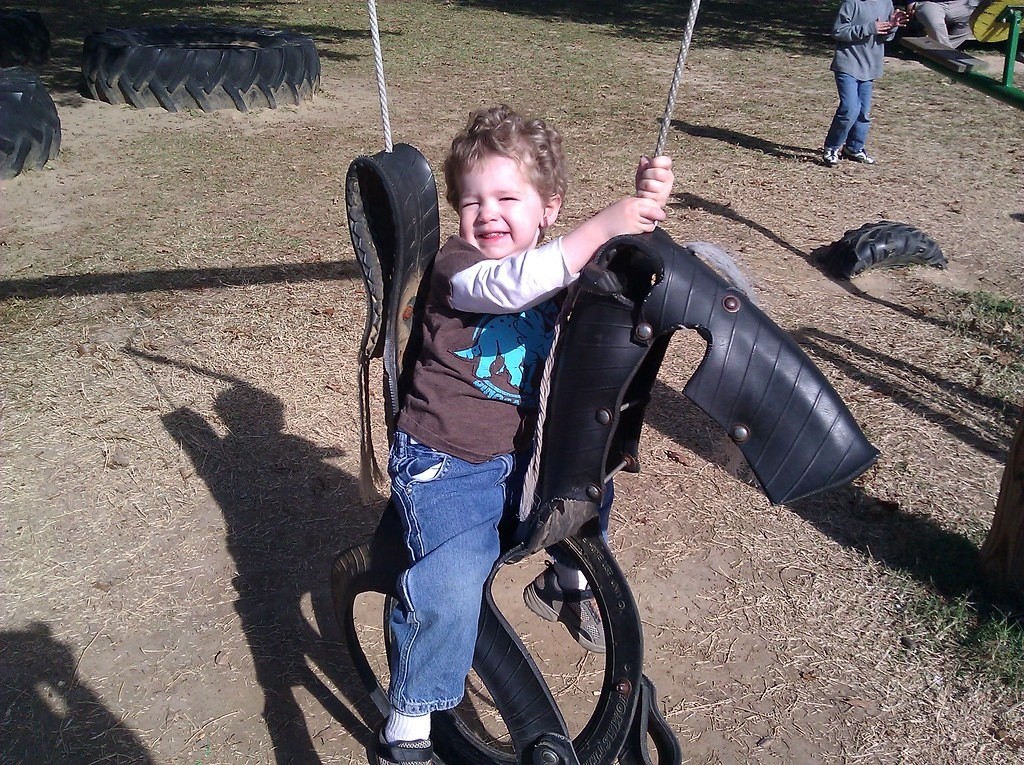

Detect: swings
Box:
350 0 886 765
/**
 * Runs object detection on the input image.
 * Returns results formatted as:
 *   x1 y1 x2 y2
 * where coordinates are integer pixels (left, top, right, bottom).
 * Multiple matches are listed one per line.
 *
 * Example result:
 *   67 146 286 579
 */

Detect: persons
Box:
906 0 975 49
823 0 909 164
374 104 673 765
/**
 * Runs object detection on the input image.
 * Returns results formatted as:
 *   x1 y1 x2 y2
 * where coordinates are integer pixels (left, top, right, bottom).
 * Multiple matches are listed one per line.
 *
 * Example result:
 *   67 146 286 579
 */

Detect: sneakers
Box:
523 559 606 653
366 713 444 765
841 144 874 164
822 144 840 167
